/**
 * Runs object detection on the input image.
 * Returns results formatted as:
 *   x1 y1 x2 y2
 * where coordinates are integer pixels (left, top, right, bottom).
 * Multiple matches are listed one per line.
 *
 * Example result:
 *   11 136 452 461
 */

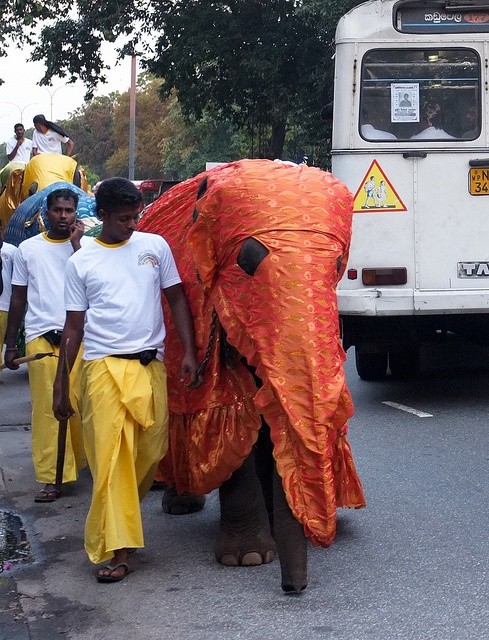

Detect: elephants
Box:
0 153 88 247
136 158 355 596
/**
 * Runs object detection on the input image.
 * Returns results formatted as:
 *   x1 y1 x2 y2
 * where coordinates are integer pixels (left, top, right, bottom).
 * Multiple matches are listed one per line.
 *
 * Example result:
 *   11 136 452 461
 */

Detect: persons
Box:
5 188 97 503
6 122 33 163
31 114 74 156
52 177 204 583
0 219 17 373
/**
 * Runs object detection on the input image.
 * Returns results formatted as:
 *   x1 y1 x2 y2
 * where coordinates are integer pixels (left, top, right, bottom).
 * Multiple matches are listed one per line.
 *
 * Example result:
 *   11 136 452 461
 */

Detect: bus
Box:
329 2 488 378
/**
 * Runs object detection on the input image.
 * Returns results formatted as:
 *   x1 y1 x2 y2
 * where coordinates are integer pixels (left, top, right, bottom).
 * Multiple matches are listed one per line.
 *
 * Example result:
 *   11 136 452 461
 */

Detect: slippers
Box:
95 562 132 582
34 489 59 502
